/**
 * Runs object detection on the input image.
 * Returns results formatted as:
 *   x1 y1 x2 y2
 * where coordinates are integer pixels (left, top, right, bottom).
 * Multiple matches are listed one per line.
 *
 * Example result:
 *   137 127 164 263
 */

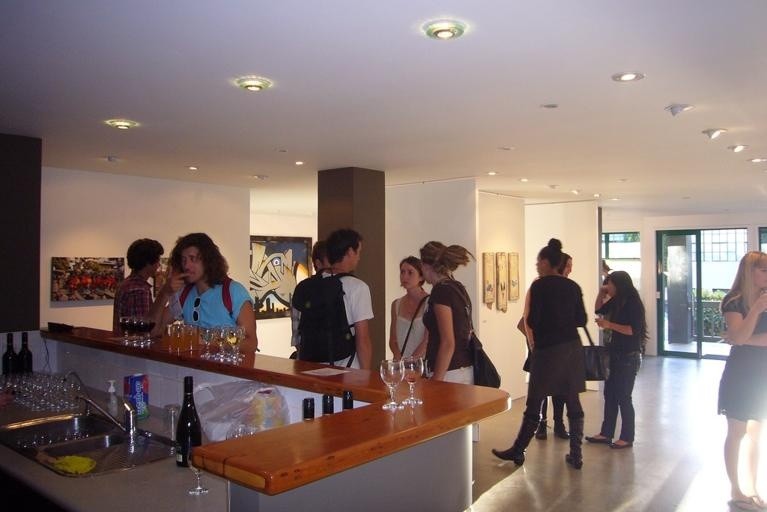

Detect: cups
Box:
176 324 193 352
166 323 179 354
161 404 182 441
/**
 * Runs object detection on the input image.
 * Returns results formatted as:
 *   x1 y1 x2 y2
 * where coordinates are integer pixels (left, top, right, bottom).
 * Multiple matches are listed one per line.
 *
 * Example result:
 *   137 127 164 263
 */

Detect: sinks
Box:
0 412 128 452
27 427 175 478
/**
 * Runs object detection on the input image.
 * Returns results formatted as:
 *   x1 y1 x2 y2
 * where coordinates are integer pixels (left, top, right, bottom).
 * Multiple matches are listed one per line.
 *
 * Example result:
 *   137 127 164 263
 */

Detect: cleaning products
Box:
106 380 119 418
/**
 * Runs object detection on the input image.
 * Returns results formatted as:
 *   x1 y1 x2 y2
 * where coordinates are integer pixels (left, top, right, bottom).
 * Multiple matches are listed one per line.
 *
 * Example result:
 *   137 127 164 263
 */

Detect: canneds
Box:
123 373 149 421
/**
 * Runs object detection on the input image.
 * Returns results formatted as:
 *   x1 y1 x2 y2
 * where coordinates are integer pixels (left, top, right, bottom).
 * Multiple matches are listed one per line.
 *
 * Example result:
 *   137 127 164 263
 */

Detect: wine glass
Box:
199 324 246 364
401 356 425 407
760 289 767 312
0 372 83 411
379 358 405 411
594 313 605 330
117 314 157 345
186 449 210 496
226 422 260 442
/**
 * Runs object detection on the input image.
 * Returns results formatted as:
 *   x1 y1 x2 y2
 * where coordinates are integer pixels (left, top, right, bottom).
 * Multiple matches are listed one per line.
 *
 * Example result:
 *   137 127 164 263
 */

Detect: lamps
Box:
237 78 271 92
110 122 136 130
671 105 684 117
426 23 464 41
708 130 720 139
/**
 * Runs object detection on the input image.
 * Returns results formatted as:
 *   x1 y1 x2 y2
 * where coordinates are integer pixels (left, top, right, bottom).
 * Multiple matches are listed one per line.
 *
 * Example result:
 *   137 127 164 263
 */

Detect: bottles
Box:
322 394 334 416
343 391 353 411
303 398 315 421
2 333 17 375
18 331 33 377
175 376 201 468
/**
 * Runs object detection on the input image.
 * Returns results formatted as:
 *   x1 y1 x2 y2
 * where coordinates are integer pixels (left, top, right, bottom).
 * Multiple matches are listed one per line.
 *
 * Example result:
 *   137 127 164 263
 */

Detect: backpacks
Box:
291 267 357 367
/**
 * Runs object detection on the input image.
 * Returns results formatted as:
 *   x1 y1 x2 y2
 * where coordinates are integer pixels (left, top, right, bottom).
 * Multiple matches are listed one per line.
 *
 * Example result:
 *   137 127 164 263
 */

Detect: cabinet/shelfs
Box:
0 133 43 333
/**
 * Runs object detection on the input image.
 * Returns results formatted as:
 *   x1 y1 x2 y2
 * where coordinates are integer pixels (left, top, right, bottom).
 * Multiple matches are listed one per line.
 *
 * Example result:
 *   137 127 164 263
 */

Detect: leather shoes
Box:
610 439 633 448
585 435 612 444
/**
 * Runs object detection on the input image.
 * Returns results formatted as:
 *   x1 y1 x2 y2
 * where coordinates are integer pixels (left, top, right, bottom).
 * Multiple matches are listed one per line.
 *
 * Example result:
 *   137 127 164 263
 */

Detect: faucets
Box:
77 394 137 454
63 370 90 416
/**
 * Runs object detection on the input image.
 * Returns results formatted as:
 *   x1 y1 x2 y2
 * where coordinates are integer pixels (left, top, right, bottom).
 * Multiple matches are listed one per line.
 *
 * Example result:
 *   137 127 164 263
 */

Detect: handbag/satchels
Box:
582 324 611 381
469 330 501 388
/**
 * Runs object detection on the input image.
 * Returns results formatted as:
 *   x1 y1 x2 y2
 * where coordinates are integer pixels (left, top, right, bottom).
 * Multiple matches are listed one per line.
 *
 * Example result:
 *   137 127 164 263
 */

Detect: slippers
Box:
729 499 758 510
751 494 766 508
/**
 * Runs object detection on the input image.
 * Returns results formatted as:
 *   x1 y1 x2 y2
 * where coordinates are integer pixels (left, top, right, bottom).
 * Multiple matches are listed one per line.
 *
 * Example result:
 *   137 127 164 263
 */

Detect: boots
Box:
492 418 538 467
554 421 570 439
536 419 551 439
565 419 583 470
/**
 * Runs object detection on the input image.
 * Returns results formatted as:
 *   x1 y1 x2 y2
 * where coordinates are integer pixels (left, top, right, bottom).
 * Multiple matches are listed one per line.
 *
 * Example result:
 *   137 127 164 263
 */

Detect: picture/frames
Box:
508 252 521 302
496 251 508 313
483 252 496 310
250 235 313 319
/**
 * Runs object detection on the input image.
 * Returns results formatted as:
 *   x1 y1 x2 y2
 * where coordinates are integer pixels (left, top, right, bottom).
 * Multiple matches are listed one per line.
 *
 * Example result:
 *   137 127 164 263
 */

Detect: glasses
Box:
191 296 201 324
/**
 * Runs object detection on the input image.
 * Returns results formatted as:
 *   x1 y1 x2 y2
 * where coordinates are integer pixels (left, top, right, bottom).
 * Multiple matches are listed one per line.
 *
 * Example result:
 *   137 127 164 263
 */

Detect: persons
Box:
113 238 169 332
296 227 375 368
419 241 477 384
389 256 432 376
148 233 258 351
717 251 767 512
290 238 331 359
491 238 652 468
